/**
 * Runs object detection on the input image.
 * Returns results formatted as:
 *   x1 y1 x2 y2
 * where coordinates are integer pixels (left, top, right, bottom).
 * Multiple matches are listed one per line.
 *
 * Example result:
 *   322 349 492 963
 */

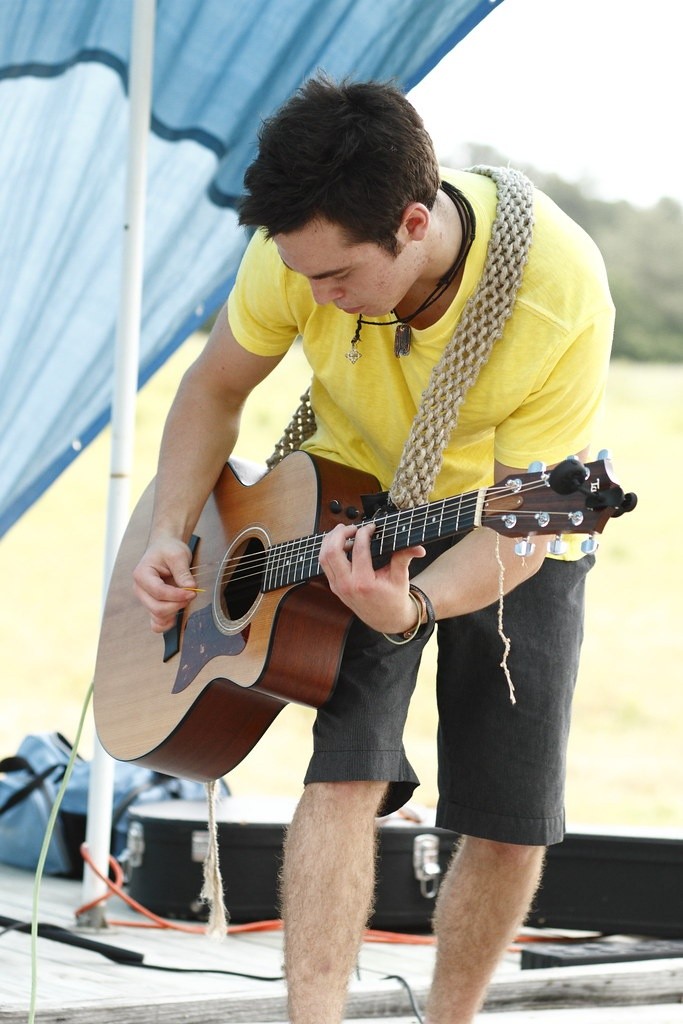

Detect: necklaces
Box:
343 177 477 364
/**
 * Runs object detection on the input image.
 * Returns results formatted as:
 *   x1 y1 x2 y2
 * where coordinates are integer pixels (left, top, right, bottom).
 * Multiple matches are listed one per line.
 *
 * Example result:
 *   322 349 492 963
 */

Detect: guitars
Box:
84 440 641 785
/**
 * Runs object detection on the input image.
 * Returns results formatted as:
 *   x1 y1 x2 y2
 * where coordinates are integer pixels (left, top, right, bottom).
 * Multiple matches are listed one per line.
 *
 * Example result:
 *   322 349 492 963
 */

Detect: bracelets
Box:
383 581 434 646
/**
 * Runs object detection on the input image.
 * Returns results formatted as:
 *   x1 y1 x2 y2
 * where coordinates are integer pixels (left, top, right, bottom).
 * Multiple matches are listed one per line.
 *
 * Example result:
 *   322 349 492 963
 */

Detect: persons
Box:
132 73 618 1023
